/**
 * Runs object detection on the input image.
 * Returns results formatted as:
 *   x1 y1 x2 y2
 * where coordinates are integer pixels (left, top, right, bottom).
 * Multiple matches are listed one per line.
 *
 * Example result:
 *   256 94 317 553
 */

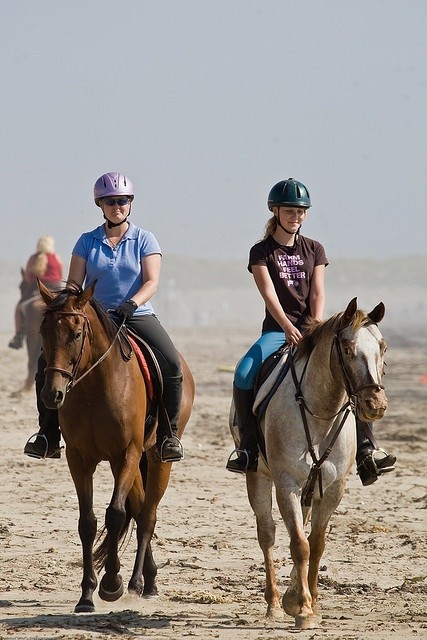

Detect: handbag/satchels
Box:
31 253 48 275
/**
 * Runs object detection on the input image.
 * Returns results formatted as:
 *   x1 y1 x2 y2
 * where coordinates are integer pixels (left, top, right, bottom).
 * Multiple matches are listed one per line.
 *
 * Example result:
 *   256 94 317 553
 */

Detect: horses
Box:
37 279 195 612
229 297 388 627
12 268 59 399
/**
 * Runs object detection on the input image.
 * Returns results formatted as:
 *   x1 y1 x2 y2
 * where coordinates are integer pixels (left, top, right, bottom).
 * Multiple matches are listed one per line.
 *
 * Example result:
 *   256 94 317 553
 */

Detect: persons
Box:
8 236 63 349
23 173 183 462
225 177 396 485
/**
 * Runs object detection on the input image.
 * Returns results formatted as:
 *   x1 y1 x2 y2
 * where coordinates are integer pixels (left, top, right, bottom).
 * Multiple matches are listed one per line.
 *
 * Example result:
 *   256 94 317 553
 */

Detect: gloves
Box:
115 300 138 321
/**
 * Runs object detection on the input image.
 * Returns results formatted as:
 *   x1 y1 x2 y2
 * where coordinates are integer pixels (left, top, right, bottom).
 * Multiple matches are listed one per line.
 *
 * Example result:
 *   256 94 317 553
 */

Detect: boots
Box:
24 372 64 459
355 416 396 485
156 374 184 461
227 382 259 473
9 333 23 348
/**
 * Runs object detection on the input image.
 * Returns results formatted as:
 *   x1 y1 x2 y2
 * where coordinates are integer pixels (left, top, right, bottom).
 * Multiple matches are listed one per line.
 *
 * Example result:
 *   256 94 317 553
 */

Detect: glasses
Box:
105 198 128 206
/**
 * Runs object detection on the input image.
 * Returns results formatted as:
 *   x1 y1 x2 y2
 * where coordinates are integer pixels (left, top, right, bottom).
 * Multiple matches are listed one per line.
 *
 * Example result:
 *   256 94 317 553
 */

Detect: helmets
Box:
94 172 134 207
268 178 312 211
